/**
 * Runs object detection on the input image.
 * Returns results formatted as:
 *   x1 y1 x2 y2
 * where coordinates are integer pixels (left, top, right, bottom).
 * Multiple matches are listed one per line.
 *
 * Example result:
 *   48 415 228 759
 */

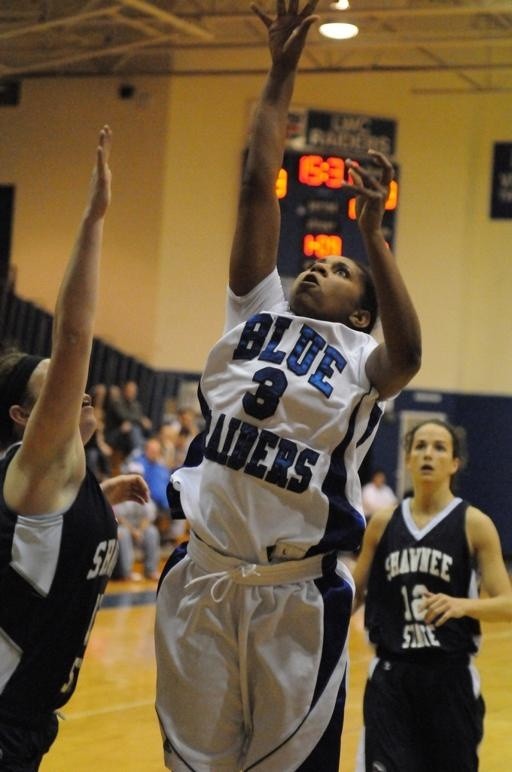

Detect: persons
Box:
154 0 424 771
0 125 150 772
362 471 397 518
352 420 511 772
83 380 204 582
